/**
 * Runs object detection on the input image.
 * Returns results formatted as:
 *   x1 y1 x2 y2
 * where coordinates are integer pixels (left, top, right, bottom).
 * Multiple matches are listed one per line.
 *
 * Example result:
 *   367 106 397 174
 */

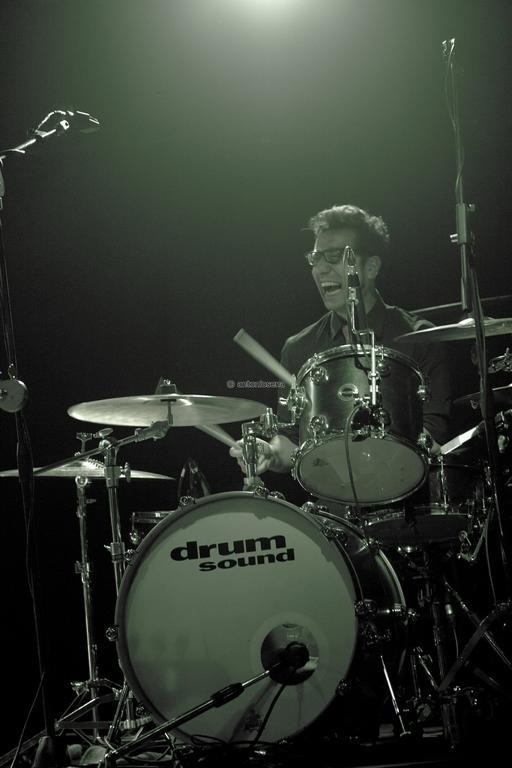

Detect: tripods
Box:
384 108 512 752
0 422 186 768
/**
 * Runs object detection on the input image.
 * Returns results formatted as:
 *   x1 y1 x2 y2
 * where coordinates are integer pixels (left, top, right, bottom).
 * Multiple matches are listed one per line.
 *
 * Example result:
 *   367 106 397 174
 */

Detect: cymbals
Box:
0 461 173 483
390 316 512 346
67 394 271 434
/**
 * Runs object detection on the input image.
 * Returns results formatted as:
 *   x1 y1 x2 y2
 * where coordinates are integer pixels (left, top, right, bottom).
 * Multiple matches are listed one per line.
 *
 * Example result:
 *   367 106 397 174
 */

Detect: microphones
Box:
280 641 309 670
189 459 210 498
347 249 367 336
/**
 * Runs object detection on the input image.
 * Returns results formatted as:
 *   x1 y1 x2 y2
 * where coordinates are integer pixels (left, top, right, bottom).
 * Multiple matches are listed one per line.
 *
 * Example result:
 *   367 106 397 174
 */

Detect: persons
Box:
228 204 453 482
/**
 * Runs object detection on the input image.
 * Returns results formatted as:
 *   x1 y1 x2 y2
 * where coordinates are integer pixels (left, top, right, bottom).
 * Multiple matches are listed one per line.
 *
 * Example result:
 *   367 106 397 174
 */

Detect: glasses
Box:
304 247 361 266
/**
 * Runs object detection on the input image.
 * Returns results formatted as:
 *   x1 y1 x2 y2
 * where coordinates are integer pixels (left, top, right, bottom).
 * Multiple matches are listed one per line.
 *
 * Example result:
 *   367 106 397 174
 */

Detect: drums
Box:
284 343 431 510
107 490 419 752
359 454 474 548
129 507 182 559
